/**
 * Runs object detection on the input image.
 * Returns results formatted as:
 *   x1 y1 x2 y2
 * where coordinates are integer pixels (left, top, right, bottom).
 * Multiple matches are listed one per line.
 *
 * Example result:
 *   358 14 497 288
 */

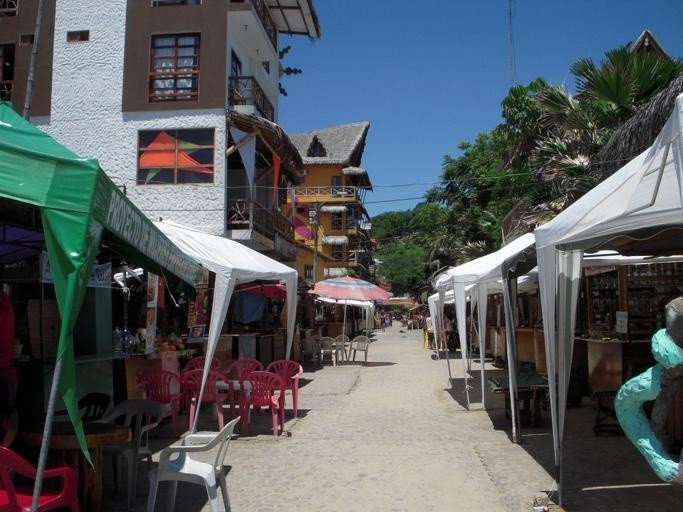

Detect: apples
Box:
176 344 184 351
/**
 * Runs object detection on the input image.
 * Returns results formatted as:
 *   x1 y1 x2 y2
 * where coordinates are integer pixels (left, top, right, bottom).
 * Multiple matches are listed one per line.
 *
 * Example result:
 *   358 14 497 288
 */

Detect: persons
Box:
380 310 453 352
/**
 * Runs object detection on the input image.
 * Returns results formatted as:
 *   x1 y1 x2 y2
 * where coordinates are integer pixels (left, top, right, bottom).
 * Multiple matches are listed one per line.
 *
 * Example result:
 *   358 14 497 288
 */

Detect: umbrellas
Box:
304 274 391 348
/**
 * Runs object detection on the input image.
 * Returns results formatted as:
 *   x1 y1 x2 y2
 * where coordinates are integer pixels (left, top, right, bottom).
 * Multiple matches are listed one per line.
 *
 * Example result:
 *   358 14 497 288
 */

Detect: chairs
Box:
54 391 114 436
307 332 370 364
1 447 80 511
94 399 163 492
584 364 635 440
147 416 241 512
137 356 304 438
2 412 19 447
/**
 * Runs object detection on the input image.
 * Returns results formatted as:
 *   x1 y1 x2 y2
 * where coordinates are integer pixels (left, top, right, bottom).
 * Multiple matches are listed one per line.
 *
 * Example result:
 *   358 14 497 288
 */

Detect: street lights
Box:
310 189 347 281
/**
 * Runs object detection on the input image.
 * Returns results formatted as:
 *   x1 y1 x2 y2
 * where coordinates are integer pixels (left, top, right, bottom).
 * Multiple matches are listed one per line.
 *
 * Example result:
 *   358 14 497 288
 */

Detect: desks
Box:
484 368 550 427
25 423 132 510
